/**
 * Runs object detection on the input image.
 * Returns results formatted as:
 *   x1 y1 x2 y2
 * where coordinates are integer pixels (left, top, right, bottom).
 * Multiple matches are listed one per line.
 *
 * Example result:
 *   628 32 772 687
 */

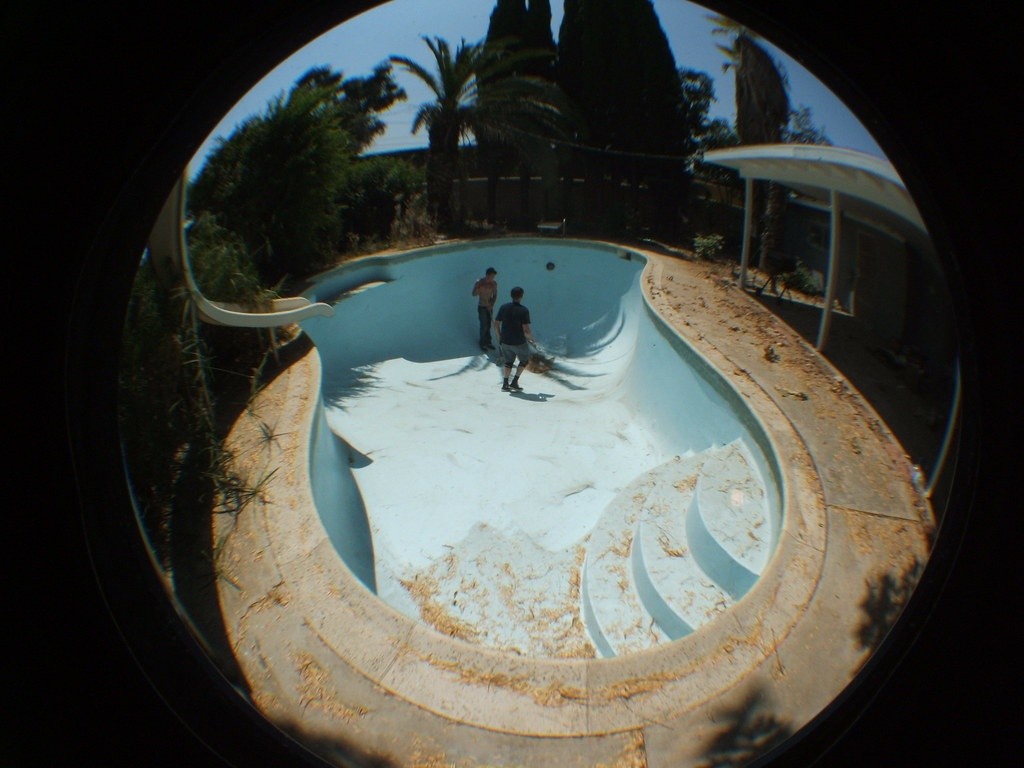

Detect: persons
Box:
472 267 497 351
494 287 536 392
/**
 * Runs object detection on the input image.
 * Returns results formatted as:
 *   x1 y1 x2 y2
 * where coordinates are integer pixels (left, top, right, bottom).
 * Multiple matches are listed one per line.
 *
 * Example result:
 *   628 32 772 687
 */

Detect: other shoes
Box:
480 345 488 353
487 343 497 350
502 384 509 391
510 381 523 391
